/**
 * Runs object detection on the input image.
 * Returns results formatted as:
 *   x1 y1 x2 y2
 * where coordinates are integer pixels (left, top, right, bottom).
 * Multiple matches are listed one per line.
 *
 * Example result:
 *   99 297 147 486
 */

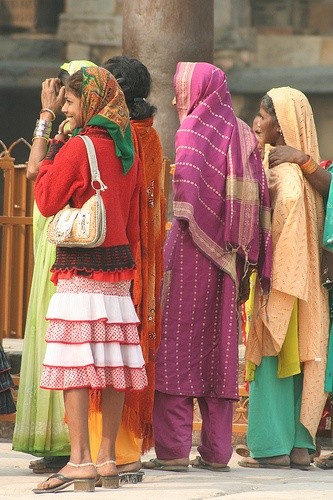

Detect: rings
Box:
58 131 62 134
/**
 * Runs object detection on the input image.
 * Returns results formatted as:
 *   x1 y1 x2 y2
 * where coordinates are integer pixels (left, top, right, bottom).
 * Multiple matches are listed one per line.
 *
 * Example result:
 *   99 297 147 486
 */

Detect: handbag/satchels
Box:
47 135 107 249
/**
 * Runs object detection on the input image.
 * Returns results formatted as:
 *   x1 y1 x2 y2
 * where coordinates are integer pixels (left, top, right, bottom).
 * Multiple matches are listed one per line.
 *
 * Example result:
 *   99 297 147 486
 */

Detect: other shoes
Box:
313 452 333 469
29 455 70 472
236 445 250 455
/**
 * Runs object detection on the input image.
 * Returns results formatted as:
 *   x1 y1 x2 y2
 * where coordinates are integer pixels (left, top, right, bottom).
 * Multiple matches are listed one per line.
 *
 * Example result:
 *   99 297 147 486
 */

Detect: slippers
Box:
113 472 137 484
191 456 231 471
238 457 290 469
134 471 144 482
288 462 315 470
141 459 189 471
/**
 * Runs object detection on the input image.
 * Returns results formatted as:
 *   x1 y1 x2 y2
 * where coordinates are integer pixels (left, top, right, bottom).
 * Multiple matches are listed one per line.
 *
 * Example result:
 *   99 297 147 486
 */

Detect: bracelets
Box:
40 109 56 121
32 119 52 143
43 139 64 159
300 155 319 175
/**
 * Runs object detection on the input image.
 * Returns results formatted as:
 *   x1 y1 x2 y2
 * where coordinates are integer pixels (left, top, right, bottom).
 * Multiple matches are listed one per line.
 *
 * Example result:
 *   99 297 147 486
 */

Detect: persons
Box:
32 65 148 494
10 60 100 474
144 62 273 472
94 56 167 486
237 86 333 470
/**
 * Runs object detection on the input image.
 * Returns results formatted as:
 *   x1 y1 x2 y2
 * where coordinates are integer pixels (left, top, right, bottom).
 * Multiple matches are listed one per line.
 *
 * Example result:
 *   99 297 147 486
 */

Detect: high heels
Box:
93 474 119 489
32 474 94 493
